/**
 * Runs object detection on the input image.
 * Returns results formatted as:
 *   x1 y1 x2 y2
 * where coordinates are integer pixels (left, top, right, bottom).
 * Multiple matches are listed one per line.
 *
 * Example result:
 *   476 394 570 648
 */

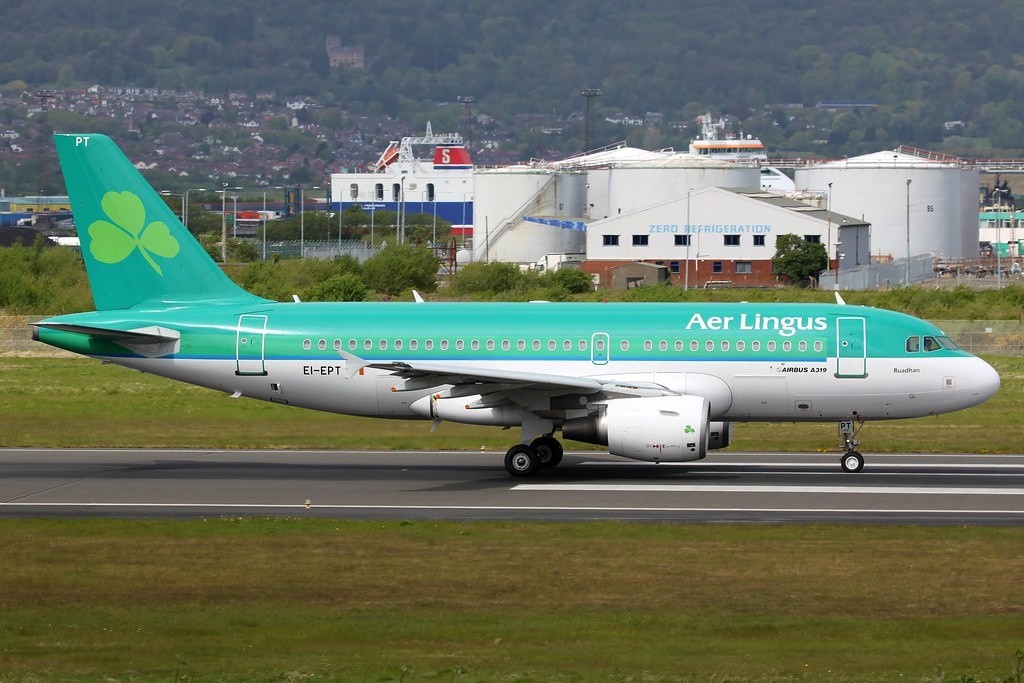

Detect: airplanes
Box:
27 133 1003 478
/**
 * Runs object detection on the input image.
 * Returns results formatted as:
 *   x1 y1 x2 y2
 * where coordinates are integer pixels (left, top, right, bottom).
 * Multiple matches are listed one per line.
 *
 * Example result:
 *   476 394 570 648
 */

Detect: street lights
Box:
684 187 694 292
221 186 243 261
401 176 405 247
462 179 466 250
906 179 913 289
826 181 833 271
184 188 207 229
